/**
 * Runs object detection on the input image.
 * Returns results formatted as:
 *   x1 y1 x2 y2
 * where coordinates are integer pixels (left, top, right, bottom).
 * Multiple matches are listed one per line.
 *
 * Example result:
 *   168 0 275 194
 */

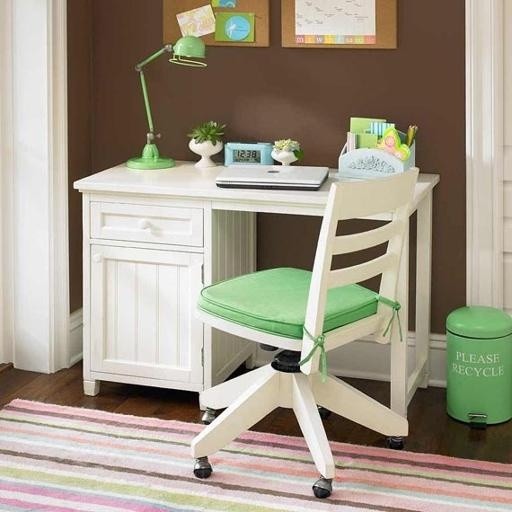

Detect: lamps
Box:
126 35 208 170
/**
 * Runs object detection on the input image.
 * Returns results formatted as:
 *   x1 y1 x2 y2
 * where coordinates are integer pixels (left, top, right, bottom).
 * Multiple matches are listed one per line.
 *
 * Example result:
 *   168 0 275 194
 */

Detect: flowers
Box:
273 138 304 160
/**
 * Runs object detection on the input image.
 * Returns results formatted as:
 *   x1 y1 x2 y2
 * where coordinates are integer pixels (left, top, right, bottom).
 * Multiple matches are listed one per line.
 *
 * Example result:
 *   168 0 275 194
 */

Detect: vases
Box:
271 150 297 165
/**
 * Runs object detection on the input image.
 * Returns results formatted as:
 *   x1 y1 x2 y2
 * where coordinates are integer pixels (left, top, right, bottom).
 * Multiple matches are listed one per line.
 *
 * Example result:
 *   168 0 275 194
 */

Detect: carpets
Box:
0 398 512 512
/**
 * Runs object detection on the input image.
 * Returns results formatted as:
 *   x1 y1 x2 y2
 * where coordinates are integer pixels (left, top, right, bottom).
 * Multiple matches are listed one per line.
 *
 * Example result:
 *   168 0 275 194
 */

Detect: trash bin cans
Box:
446 306 512 429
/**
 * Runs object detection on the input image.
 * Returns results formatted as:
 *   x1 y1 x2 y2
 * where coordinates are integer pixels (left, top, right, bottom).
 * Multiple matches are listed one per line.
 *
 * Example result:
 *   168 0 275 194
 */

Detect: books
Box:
345 116 396 153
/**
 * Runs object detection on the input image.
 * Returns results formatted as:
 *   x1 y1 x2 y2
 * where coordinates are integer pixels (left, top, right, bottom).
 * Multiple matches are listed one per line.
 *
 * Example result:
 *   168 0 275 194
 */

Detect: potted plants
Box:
187 120 227 168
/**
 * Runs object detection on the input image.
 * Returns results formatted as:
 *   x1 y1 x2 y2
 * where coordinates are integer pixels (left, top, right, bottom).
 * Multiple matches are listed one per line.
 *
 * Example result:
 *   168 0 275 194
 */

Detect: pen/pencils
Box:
407 125 418 148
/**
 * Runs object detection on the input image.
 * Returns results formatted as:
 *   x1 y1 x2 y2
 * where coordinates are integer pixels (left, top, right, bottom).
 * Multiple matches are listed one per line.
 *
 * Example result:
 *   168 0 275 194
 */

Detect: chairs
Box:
189 167 420 498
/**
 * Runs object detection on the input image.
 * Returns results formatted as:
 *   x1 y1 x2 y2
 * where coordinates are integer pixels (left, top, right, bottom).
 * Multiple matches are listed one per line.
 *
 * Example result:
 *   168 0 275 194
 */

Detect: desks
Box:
73 161 440 418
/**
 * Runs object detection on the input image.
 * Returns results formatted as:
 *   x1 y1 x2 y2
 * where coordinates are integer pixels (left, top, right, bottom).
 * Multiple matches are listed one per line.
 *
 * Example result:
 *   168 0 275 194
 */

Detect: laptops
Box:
216 162 329 191
